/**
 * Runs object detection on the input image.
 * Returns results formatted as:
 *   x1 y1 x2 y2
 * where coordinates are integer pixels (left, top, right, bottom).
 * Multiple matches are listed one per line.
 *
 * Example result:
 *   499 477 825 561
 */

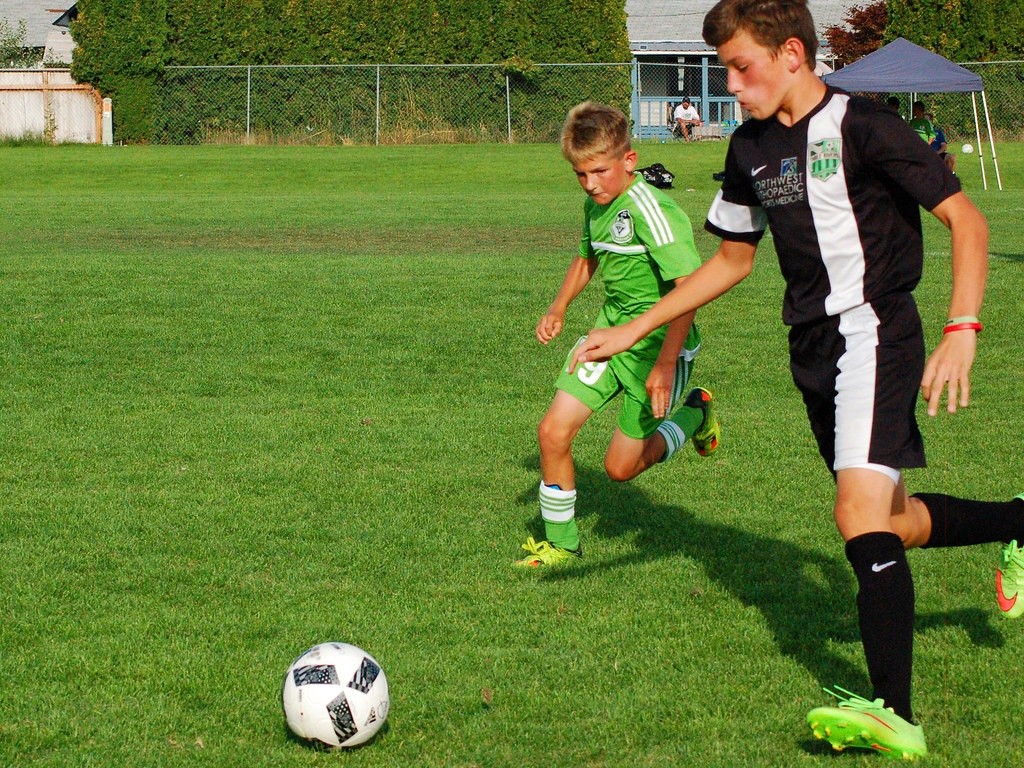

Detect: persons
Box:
887 97 901 117
570 0 1024 759
923 114 956 176
910 101 935 145
673 96 703 143
510 101 719 569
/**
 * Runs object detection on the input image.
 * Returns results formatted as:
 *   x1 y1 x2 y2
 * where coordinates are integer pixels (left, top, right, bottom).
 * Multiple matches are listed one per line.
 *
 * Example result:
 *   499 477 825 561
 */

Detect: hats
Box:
682 98 690 103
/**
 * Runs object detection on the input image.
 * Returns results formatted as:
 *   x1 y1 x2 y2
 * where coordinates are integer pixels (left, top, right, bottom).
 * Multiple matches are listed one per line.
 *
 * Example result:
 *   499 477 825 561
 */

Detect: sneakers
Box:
683 387 721 457
805 685 927 762
996 491 1024 620
510 537 584 569
953 171 965 185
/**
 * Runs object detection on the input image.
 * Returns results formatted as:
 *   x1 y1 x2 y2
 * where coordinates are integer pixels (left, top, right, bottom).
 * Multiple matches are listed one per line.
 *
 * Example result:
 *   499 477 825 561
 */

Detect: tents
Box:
821 37 1004 191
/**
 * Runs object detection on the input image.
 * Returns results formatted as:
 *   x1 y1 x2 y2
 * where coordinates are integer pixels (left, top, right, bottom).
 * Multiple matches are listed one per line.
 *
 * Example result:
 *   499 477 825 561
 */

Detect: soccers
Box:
280 641 390 752
962 143 974 154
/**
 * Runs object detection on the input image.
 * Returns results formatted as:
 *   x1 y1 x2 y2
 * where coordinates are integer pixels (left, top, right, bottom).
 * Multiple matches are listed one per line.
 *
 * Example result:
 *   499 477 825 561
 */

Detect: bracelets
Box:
943 317 982 333
